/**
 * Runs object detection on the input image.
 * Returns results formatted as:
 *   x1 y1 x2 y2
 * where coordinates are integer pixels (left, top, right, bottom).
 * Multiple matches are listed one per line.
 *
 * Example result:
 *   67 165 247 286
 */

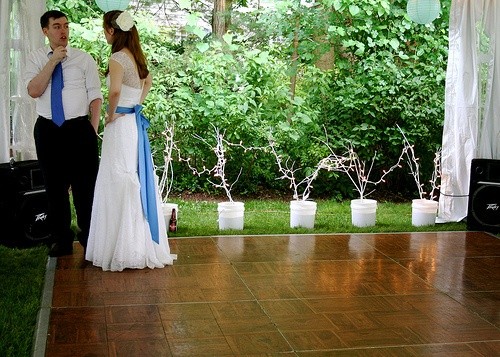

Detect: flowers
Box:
115 10 136 32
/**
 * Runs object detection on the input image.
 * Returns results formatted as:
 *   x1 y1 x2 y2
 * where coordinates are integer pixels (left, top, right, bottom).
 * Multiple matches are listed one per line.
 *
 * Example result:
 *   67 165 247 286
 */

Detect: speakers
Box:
0 160 51 249
467 159 500 233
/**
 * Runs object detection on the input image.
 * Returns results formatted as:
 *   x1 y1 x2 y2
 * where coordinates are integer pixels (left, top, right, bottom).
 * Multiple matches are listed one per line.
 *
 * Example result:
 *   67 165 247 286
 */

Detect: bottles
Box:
169 208 177 235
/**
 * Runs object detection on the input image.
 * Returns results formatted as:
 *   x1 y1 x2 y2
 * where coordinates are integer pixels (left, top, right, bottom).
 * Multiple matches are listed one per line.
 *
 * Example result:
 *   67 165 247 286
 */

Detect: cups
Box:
411 198 438 227
350 198 377 228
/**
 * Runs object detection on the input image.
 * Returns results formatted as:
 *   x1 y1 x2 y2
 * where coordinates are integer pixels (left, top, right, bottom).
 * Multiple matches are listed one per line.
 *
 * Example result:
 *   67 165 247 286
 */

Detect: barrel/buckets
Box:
290 200 317 229
217 202 245 231
162 203 178 232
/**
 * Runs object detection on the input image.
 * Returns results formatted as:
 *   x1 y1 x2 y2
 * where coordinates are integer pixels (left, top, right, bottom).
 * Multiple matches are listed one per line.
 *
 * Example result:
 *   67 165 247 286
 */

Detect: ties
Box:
50 62 65 127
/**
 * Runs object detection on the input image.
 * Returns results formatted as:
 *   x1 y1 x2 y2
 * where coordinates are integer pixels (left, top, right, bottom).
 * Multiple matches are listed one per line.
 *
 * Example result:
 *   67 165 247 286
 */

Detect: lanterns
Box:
407 0 440 24
95 0 129 12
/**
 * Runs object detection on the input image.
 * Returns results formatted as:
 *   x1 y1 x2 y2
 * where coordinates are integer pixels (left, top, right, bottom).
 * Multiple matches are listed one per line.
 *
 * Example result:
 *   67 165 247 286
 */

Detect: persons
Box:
25 10 104 257
85 10 177 271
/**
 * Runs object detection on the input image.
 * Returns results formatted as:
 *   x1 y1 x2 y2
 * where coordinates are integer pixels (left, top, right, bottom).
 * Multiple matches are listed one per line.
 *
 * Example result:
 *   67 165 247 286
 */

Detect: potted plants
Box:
253 126 356 230
150 113 179 231
396 123 453 227
184 124 245 232
314 125 409 228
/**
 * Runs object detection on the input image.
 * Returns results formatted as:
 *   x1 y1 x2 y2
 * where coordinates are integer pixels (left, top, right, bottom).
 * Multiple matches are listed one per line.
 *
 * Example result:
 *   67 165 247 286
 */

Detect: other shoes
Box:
50 244 73 256
